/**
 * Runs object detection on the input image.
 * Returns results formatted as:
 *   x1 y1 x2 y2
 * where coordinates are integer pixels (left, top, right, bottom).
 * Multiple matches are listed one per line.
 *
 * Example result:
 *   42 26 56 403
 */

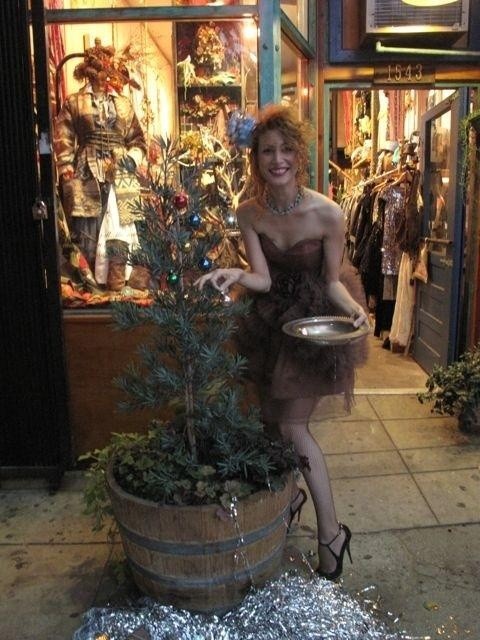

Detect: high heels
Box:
315 521 353 581
287 487 307 533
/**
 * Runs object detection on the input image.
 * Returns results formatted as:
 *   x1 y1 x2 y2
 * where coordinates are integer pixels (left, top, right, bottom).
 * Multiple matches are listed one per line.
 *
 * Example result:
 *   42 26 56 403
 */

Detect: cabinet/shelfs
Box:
171 0 246 188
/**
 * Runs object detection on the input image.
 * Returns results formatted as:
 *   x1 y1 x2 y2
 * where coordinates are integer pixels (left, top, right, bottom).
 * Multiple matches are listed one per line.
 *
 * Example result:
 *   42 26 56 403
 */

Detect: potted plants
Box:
84 134 311 623
409 341 480 437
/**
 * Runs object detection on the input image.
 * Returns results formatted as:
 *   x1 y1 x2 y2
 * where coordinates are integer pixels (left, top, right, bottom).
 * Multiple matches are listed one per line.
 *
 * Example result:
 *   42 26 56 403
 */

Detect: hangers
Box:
342 165 414 198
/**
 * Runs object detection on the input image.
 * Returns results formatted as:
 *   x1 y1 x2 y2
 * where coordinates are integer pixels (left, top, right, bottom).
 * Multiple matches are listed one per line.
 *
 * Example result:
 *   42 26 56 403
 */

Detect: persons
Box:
55 82 147 284
192 106 370 580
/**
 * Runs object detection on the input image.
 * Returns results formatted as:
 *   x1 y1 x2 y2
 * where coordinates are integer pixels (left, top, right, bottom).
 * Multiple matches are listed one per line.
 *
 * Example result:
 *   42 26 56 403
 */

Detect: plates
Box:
281 315 371 346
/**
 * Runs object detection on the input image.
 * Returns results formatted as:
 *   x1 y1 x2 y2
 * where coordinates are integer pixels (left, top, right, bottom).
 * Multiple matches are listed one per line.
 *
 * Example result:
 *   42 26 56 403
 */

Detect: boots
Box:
105 239 129 290
130 259 151 290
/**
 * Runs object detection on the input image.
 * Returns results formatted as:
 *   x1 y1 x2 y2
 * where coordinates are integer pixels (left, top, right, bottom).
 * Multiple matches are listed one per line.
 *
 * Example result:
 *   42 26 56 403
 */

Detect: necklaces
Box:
262 186 304 217
